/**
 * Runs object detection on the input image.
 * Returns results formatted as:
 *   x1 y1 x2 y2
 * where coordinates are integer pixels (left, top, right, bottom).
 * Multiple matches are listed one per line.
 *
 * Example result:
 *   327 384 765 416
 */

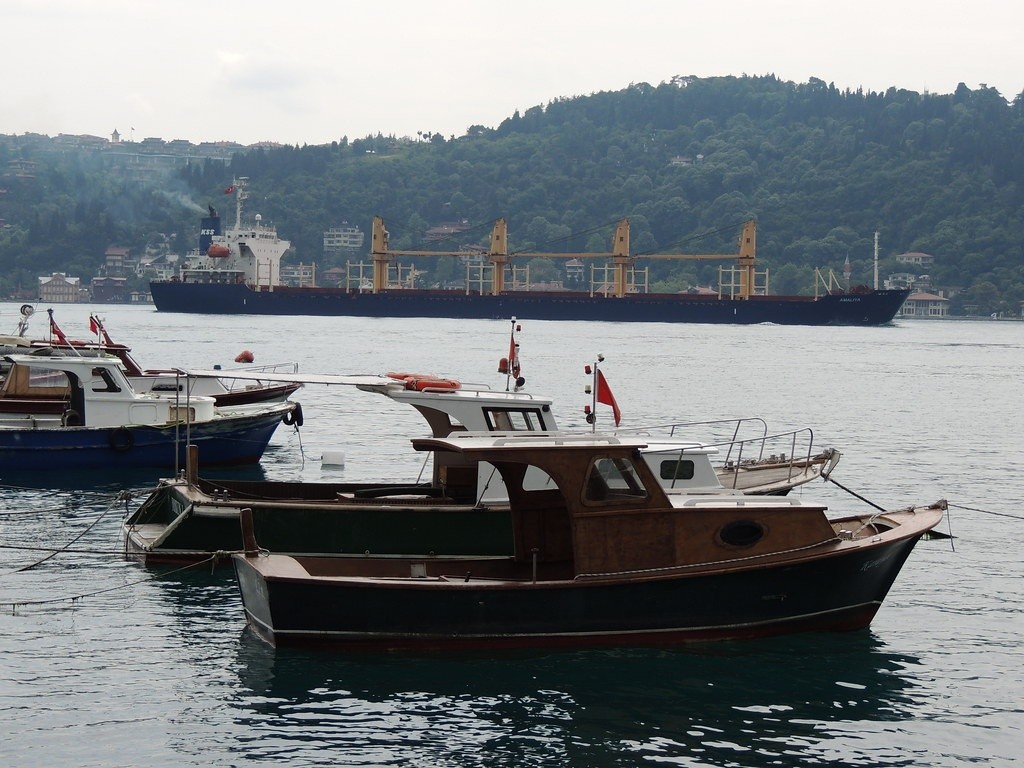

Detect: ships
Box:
149 173 914 328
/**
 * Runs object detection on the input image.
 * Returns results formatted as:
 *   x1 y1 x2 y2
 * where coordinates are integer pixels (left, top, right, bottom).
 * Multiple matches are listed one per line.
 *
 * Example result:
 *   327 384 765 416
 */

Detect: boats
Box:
0 315 299 490
0 297 304 414
122 315 843 565
230 354 948 652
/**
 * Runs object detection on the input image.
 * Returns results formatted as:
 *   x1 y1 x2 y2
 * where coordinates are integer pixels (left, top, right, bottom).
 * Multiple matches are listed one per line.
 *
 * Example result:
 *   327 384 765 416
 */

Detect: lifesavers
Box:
404 376 462 393
385 372 423 378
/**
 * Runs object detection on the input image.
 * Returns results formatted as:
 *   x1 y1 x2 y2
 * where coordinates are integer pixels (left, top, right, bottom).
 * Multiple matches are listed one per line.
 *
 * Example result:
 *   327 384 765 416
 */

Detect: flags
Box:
89 319 98 335
49 316 66 344
510 334 520 376
224 186 234 194
596 369 620 428
